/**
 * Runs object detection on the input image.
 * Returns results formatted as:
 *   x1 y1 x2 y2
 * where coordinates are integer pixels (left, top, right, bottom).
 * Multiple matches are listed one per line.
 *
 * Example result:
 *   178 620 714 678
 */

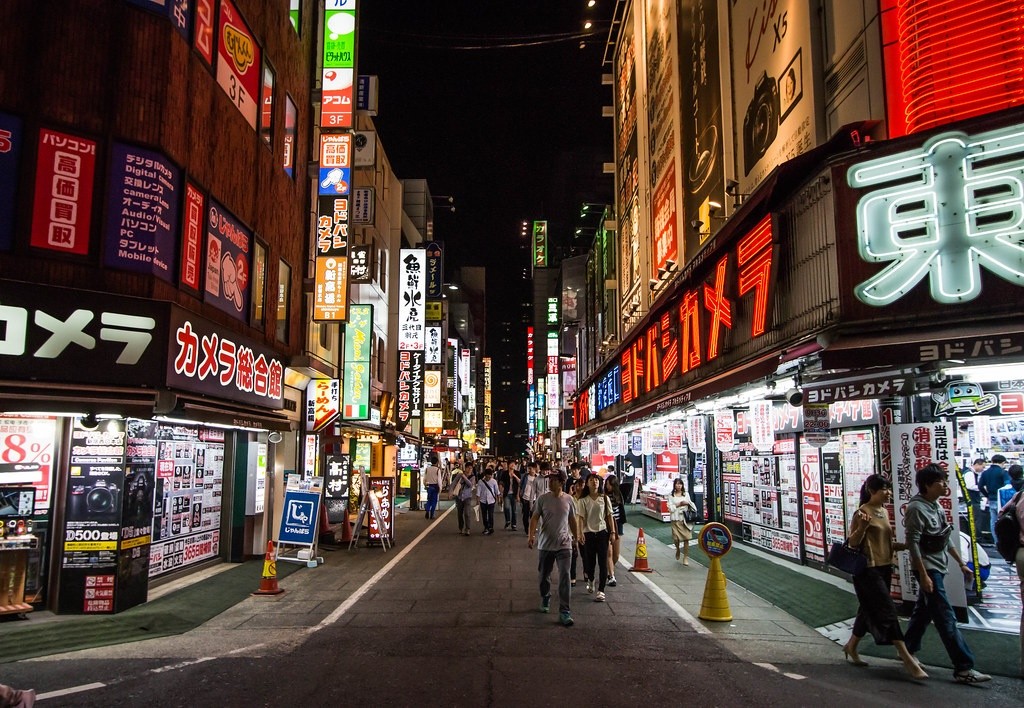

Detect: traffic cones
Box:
696 522 733 621
336 508 358 544
320 504 335 533
628 528 653 573
253 541 285 595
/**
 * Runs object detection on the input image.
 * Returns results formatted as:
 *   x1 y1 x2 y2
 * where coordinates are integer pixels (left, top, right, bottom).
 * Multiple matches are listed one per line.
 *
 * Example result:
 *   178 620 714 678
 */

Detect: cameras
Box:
70 479 121 520
743 70 779 177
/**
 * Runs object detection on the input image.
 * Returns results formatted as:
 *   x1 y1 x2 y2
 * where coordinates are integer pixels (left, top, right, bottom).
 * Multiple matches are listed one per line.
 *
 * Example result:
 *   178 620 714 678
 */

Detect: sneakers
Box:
540 598 550 613
596 591 606 602
558 613 575 626
586 578 596 594
966 583 987 596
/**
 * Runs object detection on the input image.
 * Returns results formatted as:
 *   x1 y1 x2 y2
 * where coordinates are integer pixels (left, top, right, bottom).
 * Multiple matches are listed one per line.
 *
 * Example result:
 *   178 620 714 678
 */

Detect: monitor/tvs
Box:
0 487 37 537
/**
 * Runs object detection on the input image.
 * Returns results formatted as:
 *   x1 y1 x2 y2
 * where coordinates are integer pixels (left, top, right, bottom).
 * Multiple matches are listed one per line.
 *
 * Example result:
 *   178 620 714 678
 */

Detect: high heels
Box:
903 661 930 679
842 643 868 666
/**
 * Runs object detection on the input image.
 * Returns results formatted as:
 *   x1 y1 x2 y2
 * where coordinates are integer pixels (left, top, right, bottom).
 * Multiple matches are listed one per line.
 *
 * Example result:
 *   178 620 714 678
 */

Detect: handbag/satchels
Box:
453 482 461 498
683 505 704 523
994 503 1020 567
604 516 619 541
828 536 865 575
935 523 951 555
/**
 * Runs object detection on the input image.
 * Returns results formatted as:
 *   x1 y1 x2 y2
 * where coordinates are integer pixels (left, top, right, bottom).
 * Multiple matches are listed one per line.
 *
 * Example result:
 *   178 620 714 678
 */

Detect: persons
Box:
843 474 929 680
423 454 627 625
959 455 1024 634
666 478 698 566
897 463 992 683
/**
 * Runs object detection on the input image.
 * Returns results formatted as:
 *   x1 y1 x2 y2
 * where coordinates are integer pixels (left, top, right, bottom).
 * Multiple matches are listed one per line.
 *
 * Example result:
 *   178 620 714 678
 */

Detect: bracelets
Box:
611 531 616 534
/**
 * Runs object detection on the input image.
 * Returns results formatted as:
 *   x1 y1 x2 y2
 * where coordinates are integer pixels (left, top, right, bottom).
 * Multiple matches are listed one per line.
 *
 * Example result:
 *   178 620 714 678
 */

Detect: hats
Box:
543 469 566 480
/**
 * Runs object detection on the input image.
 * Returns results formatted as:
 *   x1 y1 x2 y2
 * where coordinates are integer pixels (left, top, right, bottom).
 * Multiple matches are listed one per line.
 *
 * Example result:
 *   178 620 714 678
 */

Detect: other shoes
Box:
675 549 680 561
459 528 471 536
570 578 577 586
607 577 616 586
481 528 494 535
425 510 435 519
912 656 925 669
683 558 689 566
504 522 517 532
584 573 589 582
952 669 992 683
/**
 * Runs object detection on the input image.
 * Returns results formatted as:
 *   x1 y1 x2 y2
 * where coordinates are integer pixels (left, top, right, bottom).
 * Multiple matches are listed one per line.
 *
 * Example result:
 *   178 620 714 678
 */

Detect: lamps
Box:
623 303 648 324
708 201 729 219
658 268 670 281
650 279 661 291
726 179 750 197
666 260 681 273
691 220 710 235
